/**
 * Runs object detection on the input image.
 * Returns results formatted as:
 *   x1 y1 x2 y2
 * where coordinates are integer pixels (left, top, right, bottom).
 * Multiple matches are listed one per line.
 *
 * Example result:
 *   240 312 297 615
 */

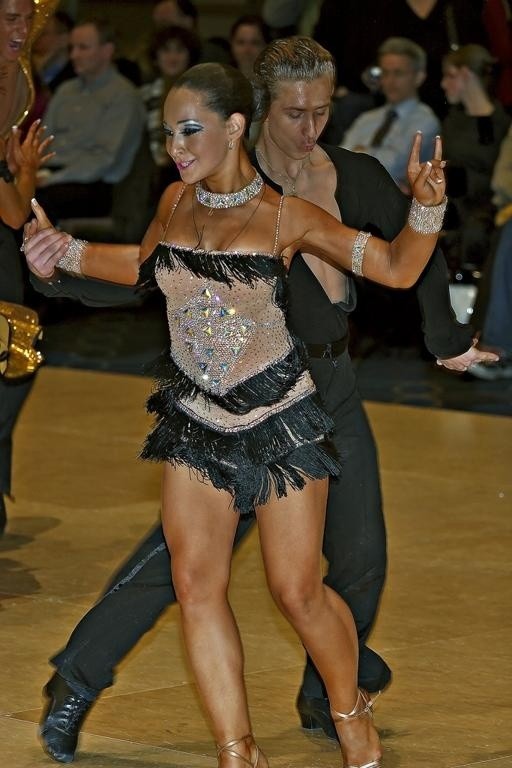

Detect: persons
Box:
19 35 501 764
22 63 449 768
0 0 58 528
24 0 512 378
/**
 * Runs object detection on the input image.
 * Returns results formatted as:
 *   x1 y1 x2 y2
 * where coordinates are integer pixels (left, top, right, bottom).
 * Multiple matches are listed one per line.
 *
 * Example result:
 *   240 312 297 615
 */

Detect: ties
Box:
371 111 398 146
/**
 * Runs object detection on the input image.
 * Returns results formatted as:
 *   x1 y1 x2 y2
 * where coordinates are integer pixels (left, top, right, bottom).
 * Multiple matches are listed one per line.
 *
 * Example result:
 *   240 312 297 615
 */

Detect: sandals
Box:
216 685 383 768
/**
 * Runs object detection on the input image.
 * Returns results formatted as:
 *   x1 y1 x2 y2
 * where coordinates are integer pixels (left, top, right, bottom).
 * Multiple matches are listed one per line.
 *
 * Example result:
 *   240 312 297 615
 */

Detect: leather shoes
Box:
40 673 93 762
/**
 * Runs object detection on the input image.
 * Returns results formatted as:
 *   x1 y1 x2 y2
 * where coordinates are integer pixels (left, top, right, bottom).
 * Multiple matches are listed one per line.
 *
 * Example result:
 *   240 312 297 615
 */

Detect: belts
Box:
306 336 346 359
